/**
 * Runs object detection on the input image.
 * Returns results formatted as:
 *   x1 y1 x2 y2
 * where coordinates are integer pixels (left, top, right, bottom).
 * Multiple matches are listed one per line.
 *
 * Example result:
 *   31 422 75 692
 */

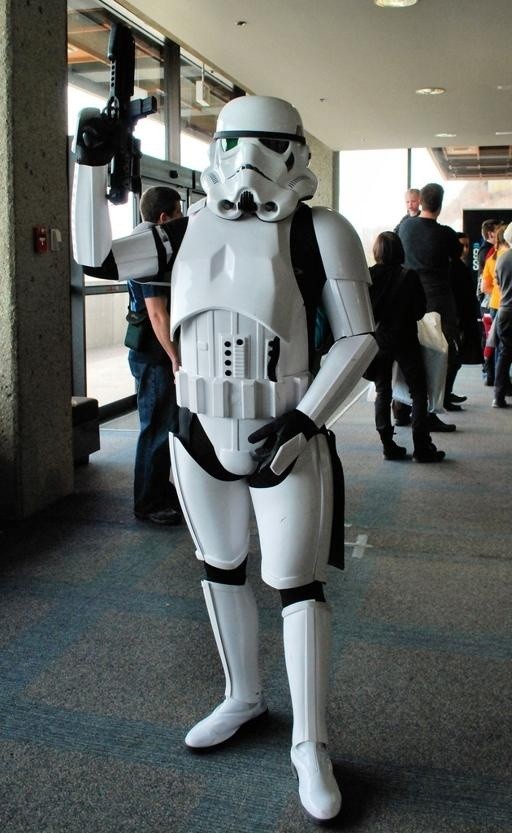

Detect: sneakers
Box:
492 399 512 408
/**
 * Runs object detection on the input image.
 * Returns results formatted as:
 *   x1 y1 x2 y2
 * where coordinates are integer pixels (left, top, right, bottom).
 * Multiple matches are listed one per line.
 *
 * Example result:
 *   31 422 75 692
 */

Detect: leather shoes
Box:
427 412 456 431
445 402 461 410
450 393 467 402
395 416 413 425
135 505 183 524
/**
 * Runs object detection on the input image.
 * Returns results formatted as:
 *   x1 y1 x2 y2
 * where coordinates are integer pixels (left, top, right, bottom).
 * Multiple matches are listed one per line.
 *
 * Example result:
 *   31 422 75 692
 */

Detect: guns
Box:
82 21 158 205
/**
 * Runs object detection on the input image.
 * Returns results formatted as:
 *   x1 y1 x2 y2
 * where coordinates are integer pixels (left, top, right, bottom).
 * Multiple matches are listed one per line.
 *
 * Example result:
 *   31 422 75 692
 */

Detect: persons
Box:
475 219 512 408
363 182 477 461
122 185 186 527
68 95 381 820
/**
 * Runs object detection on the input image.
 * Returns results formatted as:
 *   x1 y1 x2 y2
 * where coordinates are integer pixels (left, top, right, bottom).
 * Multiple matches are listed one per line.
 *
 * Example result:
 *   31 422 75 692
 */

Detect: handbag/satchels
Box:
363 332 376 380
391 310 449 413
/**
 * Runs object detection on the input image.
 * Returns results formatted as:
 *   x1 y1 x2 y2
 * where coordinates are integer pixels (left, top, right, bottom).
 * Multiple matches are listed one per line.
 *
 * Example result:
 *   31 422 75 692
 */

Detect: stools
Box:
72 396 100 468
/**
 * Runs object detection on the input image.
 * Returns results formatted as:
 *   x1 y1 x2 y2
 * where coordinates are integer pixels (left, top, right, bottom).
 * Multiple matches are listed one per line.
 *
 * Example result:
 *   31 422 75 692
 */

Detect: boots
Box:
413 429 446 462
379 425 407 456
